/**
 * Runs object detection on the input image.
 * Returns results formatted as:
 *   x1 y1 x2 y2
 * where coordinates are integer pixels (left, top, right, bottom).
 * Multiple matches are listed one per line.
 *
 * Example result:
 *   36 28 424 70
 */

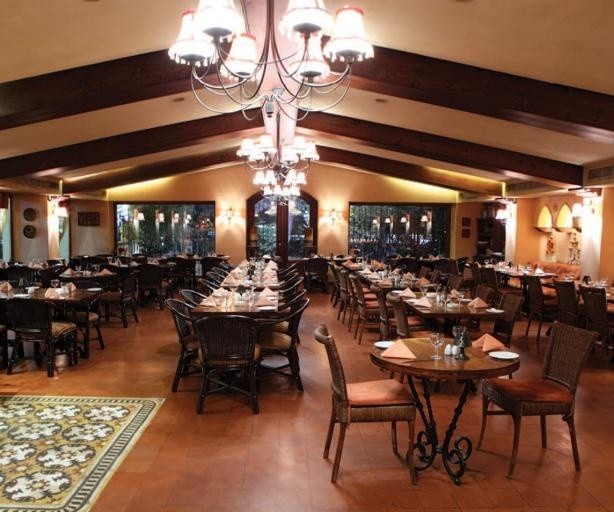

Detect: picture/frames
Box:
462 217 471 238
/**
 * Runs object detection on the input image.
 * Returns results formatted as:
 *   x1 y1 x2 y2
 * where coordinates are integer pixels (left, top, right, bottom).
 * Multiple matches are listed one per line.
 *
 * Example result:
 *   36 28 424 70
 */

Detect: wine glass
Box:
342 256 463 310
430 332 443 360
225 255 271 307
484 257 608 295
2 253 133 296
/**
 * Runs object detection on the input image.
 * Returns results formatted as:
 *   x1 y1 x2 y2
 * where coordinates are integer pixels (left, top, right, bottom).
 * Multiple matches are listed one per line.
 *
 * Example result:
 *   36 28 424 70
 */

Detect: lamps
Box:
494 197 517 220
265 201 301 216
371 209 431 223
137 212 145 221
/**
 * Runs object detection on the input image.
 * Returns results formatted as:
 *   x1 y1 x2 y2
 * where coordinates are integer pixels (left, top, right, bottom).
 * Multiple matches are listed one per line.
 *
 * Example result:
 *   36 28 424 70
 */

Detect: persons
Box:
184 235 193 252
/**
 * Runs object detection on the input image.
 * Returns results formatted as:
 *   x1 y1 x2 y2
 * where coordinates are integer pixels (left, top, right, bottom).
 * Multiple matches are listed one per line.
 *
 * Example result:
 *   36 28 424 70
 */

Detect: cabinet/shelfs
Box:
475 217 504 256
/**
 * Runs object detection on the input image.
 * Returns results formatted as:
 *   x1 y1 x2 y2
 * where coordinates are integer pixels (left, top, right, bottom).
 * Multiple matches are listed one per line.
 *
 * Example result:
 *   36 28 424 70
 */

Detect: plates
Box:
486 309 505 313
488 351 519 359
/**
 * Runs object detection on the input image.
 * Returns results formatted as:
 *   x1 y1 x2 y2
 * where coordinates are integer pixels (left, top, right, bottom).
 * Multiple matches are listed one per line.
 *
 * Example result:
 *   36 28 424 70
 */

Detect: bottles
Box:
444 343 458 356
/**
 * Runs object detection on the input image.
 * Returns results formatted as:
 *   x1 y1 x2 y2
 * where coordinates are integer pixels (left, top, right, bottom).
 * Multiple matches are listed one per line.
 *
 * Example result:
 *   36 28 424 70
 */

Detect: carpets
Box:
0 392 166 511
